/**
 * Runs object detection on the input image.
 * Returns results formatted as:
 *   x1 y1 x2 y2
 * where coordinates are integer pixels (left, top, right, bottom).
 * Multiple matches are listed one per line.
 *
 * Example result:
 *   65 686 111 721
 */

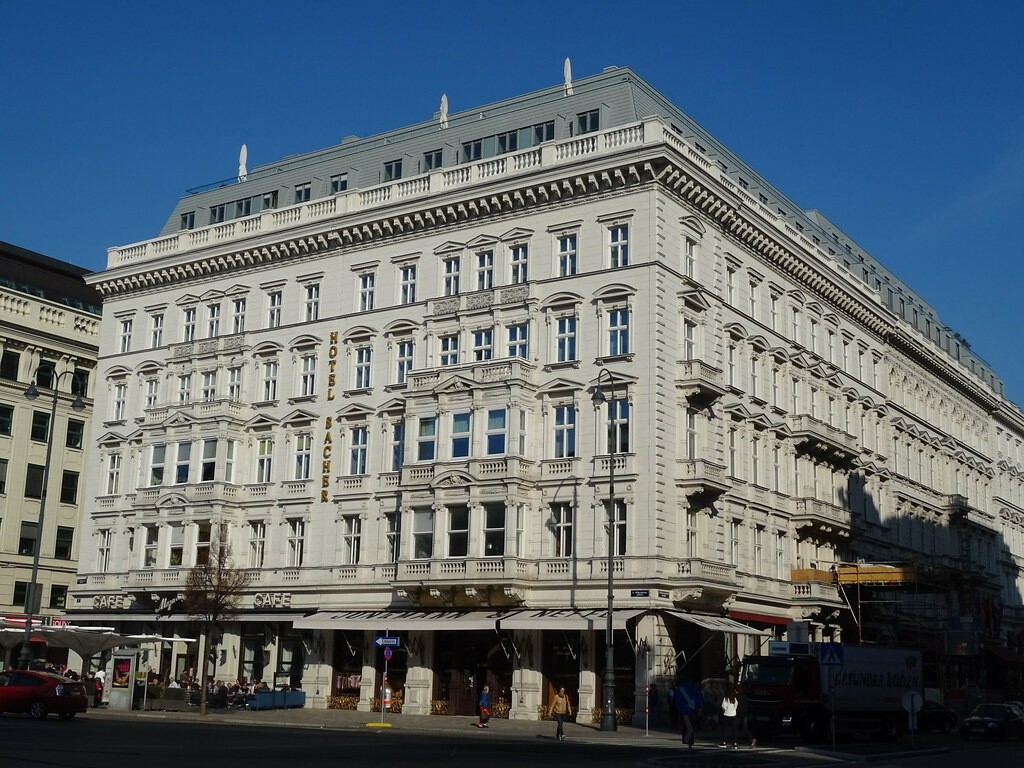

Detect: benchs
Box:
133 686 190 712
247 691 305 711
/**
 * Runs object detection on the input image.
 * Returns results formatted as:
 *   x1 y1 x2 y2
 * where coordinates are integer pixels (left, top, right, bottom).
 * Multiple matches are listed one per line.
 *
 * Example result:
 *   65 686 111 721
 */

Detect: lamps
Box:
504 587 526 601
397 589 413 601
430 588 448 602
465 587 484 600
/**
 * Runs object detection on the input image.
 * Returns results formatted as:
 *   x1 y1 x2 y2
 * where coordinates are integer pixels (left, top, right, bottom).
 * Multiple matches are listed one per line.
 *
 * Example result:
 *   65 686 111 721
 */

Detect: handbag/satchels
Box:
565 702 573 721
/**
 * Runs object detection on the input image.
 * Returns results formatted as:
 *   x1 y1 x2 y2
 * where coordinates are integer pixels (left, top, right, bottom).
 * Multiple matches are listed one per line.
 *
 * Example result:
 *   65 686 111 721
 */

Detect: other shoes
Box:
477 725 487 728
554 736 558 740
561 734 565 740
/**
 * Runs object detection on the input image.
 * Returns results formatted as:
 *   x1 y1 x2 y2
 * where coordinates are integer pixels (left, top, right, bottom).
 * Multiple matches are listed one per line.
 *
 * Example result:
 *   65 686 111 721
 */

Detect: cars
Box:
1 668 90 721
918 700 957 734
958 701 1024 741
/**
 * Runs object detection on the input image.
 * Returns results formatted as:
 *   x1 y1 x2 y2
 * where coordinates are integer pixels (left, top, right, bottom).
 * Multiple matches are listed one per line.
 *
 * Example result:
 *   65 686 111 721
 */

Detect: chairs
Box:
81 679 97 707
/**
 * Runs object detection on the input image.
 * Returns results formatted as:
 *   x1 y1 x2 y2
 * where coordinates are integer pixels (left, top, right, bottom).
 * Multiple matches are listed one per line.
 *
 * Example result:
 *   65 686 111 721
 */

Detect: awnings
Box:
292 607 648 659
660 607 775 675
981 640 1023 663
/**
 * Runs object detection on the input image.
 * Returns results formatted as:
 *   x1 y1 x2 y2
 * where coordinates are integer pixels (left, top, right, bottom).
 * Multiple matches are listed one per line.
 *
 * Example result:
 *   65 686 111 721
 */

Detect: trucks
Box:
734 640 924 746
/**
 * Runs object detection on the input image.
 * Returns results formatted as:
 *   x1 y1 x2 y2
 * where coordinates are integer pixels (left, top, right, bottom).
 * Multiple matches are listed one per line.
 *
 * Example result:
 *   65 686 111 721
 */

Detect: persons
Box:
478 686 490 728
648 683 659 700
716 681 757 749
34 662 272 710
546 685 572 741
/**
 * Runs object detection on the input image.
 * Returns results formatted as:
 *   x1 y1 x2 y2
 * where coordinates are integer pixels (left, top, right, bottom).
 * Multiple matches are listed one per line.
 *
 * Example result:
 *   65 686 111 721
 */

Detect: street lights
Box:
16 363 86 669
590 365 620 733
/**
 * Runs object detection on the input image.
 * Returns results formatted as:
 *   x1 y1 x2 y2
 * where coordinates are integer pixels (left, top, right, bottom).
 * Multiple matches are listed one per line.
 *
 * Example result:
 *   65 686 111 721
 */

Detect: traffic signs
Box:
374 635 400 647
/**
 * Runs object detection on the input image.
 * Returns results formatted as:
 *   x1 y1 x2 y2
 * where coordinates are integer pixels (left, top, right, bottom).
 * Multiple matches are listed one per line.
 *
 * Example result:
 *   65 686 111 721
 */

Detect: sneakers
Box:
733 743 738 747
718 742 726 749
749 739 757 747
